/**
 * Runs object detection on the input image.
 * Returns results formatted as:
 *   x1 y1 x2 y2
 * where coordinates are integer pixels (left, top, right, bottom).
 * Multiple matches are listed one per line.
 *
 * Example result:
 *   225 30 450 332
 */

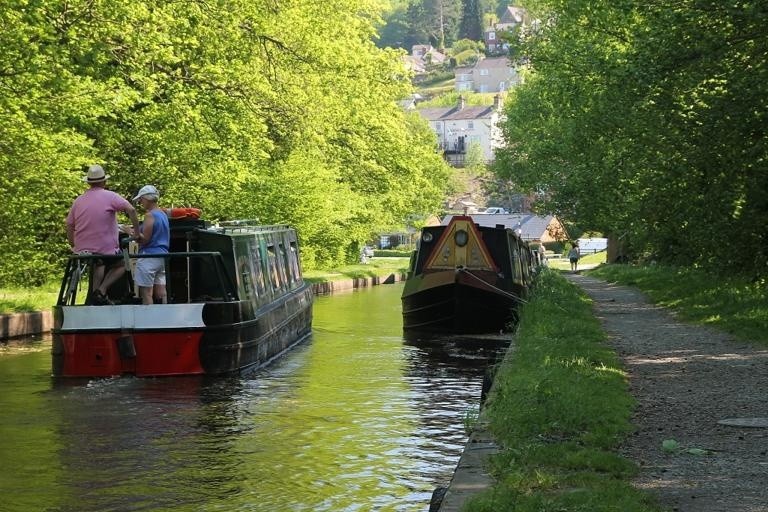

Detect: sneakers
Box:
91 289 115 305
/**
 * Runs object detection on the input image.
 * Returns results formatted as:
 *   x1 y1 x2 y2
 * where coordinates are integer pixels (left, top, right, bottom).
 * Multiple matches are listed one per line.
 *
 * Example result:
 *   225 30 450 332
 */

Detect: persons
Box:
65 163 139 306
537 242 545 265
119 184 170 305
567 243 579 274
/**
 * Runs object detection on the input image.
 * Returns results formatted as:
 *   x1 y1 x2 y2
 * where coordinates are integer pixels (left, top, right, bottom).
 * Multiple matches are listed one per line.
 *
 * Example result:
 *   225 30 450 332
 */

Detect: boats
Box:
45 210 314 383
393 212 548 345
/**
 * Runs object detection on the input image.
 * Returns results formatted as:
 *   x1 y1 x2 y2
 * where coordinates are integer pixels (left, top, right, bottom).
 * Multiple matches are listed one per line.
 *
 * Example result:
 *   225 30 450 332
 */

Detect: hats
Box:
82 165 111 183
132 184 158 201
